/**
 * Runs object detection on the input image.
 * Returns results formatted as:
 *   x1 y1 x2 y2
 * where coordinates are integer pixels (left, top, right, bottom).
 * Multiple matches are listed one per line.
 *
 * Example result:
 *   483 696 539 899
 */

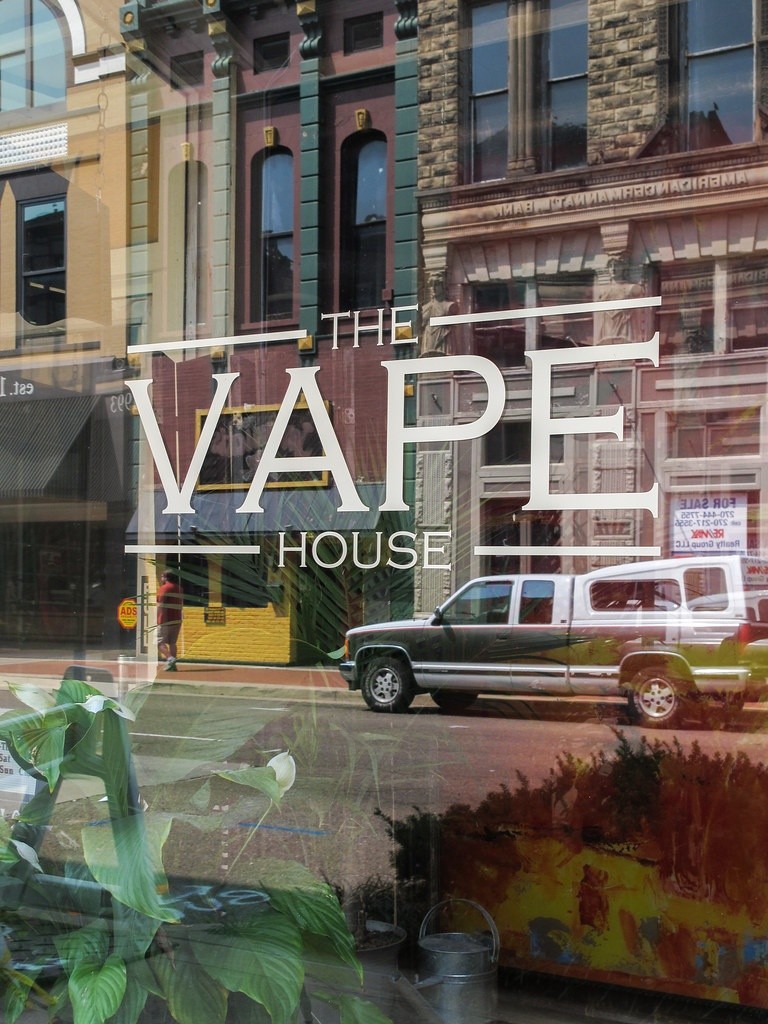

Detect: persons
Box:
595 257 644 343
418 270 459 357
156 569 182 669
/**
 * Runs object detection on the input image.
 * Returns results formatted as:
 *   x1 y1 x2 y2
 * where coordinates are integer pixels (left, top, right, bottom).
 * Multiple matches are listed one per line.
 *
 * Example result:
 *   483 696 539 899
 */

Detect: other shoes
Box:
162 658 178 672
168 665 177 672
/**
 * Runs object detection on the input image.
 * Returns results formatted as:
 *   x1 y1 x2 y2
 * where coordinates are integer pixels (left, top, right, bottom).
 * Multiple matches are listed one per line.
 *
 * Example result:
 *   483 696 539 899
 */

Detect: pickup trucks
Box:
337 557 768 730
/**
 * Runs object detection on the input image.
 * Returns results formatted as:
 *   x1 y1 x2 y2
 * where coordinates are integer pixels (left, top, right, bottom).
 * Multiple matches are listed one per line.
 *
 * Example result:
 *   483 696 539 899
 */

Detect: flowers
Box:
1 679 390 1024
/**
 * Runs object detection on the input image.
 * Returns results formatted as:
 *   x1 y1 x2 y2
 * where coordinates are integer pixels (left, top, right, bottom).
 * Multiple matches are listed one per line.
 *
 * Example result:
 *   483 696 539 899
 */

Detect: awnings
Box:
124 484 389 541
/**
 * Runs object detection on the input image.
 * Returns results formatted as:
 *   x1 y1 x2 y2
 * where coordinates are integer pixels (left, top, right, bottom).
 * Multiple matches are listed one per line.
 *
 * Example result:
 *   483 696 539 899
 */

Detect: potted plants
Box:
267 668 440 1024
